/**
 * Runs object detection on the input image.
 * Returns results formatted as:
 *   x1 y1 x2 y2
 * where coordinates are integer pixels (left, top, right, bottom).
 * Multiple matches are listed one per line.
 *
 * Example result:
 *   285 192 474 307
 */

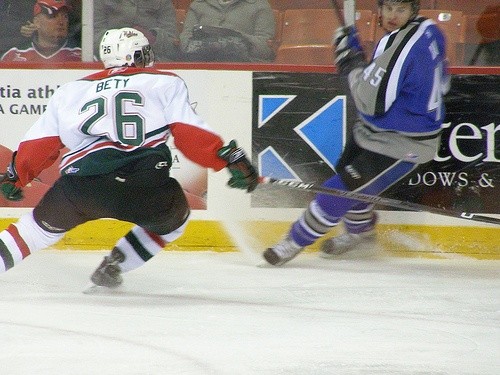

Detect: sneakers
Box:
82 245 123 297
261 234 304 267
320 229 380 260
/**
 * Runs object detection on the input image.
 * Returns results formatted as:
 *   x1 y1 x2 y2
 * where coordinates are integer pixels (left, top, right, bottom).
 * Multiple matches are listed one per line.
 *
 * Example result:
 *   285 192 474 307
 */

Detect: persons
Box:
88 0 180 64
179 0 275 64
0 27 260 289
263 0 450 267
1 0 85 62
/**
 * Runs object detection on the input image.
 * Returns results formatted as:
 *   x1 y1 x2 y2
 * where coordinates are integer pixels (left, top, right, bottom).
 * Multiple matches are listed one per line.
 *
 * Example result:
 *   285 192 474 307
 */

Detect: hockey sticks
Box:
256 175 500 224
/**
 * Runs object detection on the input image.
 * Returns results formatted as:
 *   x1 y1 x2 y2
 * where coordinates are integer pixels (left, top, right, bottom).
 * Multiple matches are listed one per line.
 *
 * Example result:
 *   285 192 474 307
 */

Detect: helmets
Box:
99 26 157 68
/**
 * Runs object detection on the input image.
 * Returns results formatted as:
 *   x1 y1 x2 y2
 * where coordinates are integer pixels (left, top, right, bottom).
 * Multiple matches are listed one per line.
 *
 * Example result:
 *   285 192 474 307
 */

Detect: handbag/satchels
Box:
186 27 251 62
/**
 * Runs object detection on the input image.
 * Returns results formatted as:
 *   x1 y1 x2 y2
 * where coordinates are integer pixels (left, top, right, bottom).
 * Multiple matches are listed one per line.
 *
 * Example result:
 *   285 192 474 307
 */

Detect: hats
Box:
34 0 73 15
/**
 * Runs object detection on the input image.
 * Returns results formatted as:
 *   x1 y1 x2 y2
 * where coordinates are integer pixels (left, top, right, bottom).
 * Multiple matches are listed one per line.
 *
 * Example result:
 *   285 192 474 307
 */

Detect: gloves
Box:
334 23 368 75
217 139 257 192
0 151 26 203
